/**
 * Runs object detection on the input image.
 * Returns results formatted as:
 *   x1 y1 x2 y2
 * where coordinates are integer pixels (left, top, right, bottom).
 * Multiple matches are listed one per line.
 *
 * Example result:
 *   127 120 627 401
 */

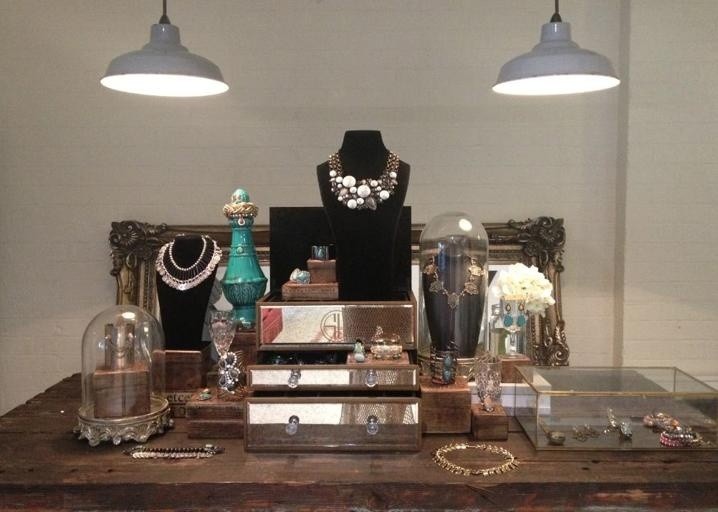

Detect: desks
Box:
2 373 715 509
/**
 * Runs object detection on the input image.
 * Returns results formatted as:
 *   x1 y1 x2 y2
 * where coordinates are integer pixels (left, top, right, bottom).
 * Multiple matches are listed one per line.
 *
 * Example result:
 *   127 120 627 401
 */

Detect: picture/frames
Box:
111 211 573 366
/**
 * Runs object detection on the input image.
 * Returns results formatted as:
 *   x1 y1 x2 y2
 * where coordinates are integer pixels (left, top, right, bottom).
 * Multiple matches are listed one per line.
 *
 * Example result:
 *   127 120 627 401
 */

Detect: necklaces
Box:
156 234 224 292
431 437 520 478
122 444 226 459
326 151 398 214
422 253 485 312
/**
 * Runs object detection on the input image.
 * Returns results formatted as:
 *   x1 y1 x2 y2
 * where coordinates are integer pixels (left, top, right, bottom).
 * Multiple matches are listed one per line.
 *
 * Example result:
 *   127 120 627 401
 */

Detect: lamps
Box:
487 1 621 99
98 0 230 100
